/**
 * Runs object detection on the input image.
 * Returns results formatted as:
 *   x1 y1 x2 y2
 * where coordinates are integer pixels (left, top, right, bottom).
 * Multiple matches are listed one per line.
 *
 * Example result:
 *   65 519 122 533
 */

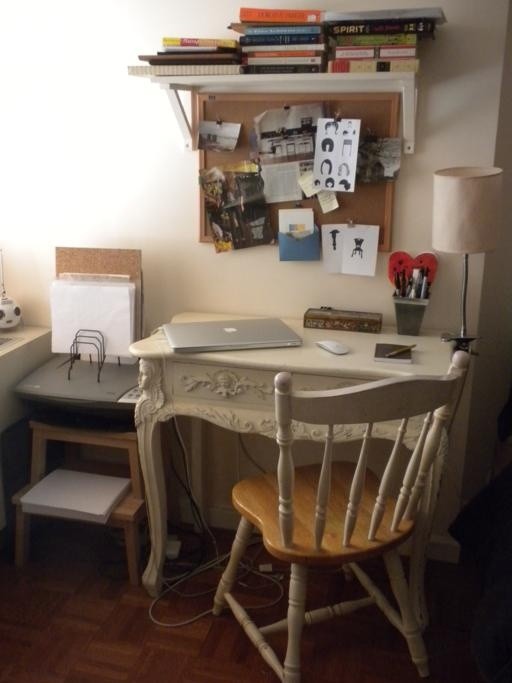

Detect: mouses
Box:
315 340 350 355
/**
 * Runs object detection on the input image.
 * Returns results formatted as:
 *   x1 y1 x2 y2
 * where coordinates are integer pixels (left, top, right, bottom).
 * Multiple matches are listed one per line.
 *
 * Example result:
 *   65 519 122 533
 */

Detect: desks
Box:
128 311 468 635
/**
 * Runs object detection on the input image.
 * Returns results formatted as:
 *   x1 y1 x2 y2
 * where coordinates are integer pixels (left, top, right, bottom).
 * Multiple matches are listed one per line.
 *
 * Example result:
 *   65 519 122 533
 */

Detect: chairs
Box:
212 351 470 683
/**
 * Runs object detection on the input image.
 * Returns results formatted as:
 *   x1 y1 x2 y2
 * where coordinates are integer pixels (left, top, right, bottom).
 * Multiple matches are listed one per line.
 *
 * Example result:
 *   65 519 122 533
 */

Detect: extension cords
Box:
254 568 281 580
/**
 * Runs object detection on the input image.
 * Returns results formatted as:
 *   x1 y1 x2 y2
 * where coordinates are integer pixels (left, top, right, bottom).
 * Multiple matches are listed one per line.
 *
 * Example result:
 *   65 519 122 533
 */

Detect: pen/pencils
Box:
385 344 417 358
394 266 429 299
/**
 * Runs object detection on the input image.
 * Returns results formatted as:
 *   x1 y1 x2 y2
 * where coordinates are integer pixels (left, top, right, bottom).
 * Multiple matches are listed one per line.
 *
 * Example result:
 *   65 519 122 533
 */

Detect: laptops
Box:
163 317 303 354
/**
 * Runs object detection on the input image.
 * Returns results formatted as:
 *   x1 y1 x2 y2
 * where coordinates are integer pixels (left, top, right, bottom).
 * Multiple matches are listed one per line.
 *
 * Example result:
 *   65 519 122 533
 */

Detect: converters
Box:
259 563 273 572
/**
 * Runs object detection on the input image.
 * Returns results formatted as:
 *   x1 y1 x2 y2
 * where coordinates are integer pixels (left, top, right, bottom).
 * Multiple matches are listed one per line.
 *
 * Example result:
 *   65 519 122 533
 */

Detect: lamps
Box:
432 166 503 355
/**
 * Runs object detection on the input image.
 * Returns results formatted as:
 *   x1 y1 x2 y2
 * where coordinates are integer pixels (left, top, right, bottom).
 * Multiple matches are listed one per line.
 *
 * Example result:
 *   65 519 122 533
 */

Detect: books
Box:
129 38 246 76
228 7 330 72
20 470 131 524
324 7 446 71
374 344 412 363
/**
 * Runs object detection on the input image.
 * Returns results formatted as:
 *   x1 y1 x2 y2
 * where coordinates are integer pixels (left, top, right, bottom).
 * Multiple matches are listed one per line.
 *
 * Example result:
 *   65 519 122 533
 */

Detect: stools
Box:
28 418 144 500
10 486 147 589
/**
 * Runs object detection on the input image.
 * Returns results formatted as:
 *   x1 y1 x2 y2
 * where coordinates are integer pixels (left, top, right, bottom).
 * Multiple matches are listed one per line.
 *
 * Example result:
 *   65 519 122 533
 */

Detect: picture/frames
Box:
196 93 400 253
